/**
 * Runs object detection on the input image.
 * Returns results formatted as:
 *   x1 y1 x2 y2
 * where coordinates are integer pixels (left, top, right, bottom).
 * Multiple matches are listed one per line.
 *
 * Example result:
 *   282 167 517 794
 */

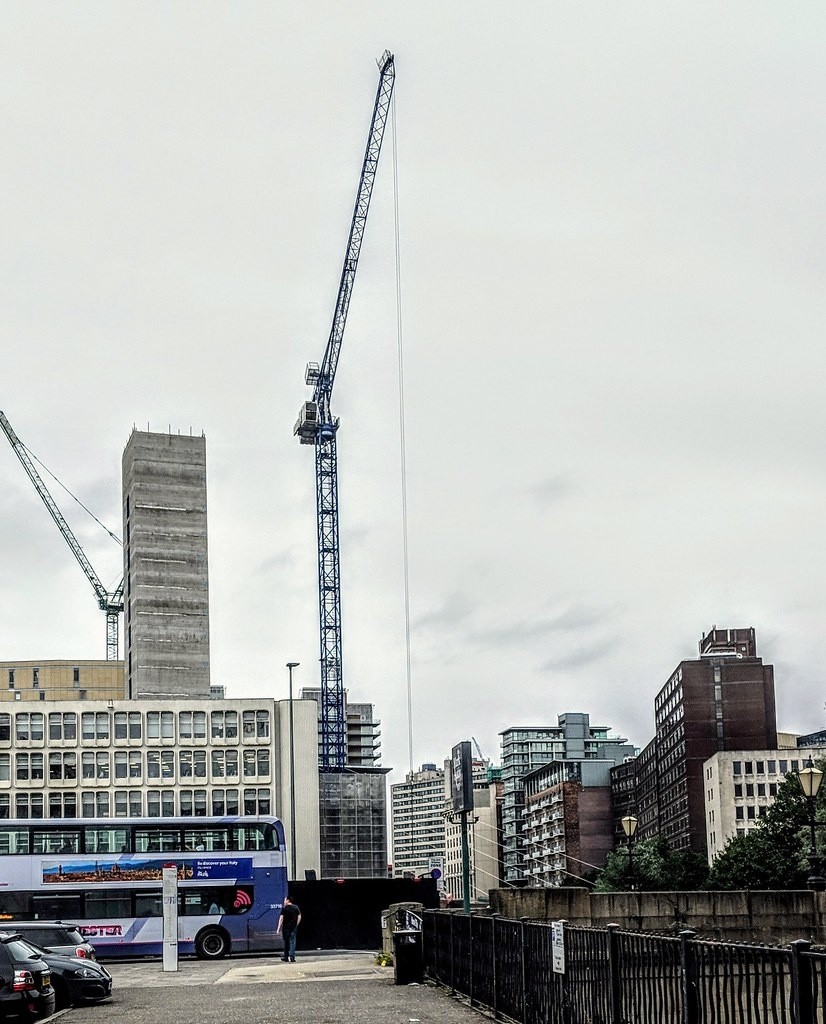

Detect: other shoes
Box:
291 959 296 962
281 957 289 961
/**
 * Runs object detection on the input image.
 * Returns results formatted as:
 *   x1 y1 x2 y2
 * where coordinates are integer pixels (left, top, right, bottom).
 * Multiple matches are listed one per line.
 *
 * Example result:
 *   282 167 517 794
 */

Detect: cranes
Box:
294 50 399 776
0 411 123 660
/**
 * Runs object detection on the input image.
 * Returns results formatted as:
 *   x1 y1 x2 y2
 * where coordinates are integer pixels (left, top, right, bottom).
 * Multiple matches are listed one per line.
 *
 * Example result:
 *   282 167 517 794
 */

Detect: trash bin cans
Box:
392 929 423 984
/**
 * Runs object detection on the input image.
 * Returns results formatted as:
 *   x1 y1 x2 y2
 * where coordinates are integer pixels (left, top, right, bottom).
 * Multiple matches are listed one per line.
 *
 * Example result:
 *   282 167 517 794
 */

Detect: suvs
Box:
0 931 56 1024
0 921 96 963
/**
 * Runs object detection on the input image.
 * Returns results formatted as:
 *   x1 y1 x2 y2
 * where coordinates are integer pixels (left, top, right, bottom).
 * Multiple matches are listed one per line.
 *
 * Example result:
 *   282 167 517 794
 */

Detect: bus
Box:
0 815 287 959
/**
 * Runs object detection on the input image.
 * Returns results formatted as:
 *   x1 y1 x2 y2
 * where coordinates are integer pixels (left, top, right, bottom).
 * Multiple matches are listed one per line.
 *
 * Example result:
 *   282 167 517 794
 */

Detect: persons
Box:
277 896 301 962
185 837 204 851
58 836 73 853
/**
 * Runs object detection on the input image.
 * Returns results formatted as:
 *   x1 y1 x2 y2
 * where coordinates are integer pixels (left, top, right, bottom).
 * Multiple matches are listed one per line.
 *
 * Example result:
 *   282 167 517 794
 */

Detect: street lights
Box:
621 813 641 892
286 661 302 880
791 754 826 890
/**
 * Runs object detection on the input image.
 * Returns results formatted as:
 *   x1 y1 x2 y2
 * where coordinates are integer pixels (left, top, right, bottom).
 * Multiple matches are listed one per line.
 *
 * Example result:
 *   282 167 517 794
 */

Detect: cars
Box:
20 938 113 999
